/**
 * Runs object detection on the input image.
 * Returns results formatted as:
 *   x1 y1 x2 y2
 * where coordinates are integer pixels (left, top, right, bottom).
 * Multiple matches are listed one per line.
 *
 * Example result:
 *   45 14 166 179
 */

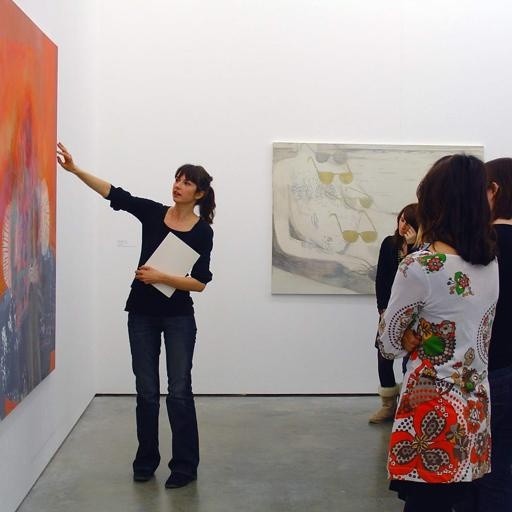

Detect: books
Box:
134 231 200 300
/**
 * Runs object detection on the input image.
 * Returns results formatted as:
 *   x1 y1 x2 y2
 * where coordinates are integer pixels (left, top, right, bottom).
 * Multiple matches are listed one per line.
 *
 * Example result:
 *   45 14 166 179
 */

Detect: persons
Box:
55 137 213 490
370 203 424 423
376 153 500 510
474 158 512 511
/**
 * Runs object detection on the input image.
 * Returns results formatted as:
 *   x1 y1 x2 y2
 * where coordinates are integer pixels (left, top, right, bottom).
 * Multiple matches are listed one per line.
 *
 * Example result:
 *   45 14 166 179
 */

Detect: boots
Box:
368 386 400 424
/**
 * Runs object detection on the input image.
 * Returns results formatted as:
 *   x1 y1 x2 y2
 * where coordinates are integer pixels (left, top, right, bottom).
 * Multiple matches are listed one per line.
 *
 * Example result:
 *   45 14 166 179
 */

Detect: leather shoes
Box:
165 472 193 488
134 469 153 481
